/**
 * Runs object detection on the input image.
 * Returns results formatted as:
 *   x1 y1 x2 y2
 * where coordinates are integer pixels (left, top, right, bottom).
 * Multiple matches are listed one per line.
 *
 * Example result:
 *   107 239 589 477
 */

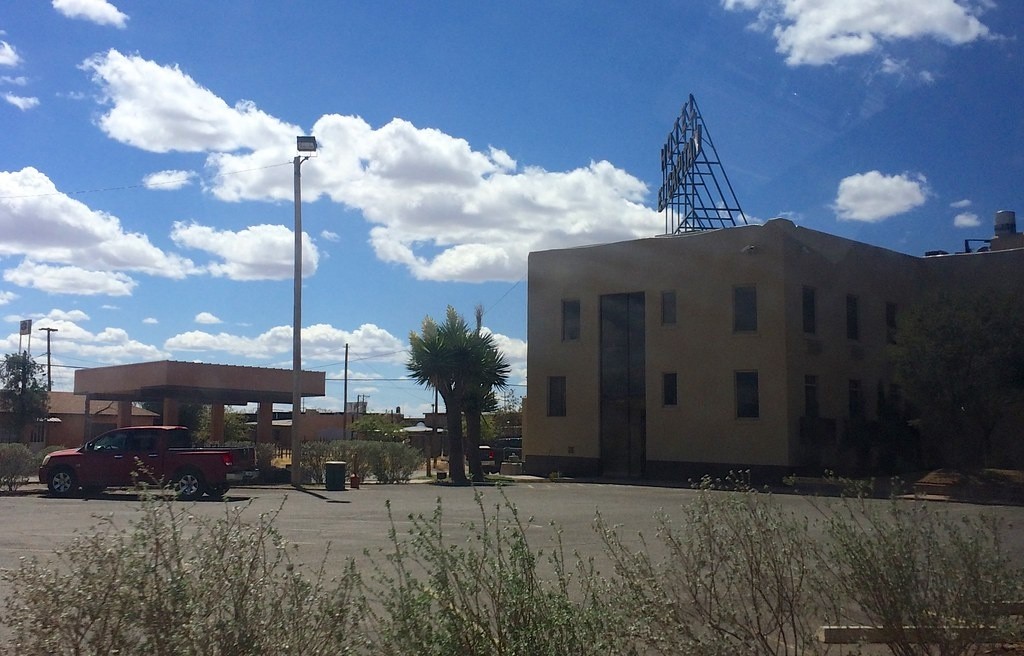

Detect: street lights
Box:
292 136 316 483
38 327 58 392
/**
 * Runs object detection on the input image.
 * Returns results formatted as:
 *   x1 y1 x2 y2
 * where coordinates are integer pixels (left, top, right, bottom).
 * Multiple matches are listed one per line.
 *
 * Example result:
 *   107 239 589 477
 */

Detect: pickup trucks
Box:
38 426 259 501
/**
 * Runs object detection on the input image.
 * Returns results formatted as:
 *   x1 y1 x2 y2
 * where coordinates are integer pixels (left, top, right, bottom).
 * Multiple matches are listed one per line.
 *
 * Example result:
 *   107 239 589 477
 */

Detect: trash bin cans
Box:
326 461 346 491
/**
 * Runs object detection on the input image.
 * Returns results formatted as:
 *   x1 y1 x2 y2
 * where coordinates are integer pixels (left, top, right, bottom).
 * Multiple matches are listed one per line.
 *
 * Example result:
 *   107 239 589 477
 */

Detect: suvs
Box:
479 446 505 474
